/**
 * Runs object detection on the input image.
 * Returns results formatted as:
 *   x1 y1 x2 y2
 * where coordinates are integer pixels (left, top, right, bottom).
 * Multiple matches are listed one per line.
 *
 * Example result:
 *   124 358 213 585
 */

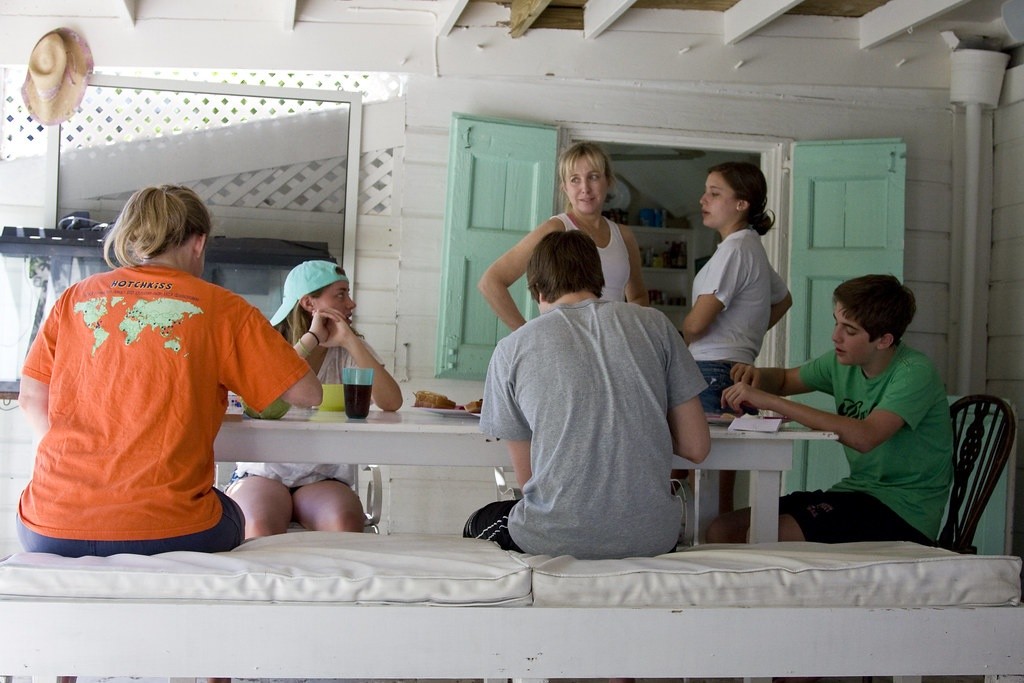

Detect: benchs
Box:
0 529 1024 680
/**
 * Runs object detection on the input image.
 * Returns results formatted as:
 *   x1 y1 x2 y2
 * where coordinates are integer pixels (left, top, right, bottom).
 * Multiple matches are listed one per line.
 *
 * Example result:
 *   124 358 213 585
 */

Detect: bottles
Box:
638 241 686 267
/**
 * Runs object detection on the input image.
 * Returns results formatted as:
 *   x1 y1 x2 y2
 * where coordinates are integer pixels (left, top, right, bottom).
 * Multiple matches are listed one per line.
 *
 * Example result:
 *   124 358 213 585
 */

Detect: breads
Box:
414 390 482 413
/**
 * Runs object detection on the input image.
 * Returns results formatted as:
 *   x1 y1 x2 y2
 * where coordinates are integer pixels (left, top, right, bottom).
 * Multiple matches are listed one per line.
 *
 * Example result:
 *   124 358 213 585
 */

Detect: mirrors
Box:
40 67 364 321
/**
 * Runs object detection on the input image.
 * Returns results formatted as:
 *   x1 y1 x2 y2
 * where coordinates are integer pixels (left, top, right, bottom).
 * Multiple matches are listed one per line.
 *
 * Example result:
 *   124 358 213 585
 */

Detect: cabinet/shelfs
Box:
626 225 696 333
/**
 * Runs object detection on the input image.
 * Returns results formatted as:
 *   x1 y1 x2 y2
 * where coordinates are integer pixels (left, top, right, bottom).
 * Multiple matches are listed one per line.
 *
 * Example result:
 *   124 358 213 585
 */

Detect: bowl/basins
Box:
316 384 344 413
237 395 290 420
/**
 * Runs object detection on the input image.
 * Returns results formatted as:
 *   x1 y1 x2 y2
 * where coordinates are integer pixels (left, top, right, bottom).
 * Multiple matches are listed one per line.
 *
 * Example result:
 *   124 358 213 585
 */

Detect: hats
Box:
268 260 349 327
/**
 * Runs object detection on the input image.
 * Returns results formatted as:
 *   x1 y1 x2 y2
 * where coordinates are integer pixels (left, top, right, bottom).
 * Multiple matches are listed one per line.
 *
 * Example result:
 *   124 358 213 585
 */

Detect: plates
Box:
705 414 791 426
410 406 480 416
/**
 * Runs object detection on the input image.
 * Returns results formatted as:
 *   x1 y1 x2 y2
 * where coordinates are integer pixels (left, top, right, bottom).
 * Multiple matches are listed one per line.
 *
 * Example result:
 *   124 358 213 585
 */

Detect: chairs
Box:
934 393 1017 555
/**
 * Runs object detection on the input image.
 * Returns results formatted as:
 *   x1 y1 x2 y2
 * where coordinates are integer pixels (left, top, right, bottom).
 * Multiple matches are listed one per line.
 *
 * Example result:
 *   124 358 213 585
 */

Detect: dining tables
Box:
211 403 840 542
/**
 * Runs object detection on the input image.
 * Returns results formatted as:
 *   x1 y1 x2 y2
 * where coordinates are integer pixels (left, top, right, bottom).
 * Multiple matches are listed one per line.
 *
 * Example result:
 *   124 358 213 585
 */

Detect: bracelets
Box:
297 338 311 356
308 330 320 346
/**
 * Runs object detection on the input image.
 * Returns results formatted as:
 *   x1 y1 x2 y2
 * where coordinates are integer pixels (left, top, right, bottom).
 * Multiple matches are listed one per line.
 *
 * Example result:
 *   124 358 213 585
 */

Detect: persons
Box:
17 179 404 559
462 229 713 565
476 140 651 331
671 163 795 513
707 274 955 548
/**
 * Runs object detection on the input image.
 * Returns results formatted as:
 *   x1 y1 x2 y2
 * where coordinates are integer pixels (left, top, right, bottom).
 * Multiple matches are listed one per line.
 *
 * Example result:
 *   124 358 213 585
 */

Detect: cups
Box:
341 366 374 419
